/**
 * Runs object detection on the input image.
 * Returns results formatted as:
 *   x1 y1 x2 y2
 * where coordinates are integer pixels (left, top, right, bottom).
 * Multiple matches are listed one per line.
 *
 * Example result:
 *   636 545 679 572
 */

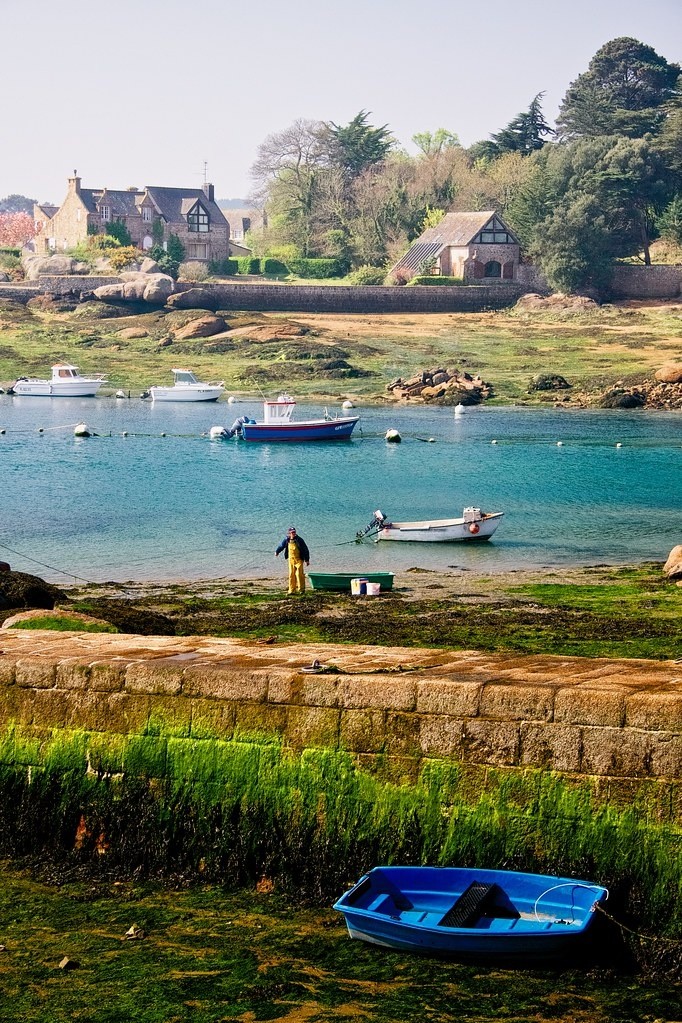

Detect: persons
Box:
275 527 310 594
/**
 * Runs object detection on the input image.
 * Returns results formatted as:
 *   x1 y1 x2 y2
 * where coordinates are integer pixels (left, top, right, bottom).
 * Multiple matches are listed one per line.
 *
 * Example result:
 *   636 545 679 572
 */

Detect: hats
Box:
289 527 296 532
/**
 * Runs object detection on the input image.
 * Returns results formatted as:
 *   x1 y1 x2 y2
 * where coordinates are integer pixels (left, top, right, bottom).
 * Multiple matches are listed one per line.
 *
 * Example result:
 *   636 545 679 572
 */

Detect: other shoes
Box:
286 593 295 596
300 592 304 594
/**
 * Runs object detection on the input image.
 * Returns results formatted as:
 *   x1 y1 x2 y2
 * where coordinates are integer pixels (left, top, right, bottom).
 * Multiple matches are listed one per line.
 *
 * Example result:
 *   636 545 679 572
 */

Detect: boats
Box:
6 361 110 397
208 392 362 441
355 505 505 543
306 569 397 592
140 368 227 403
330 864 611 955
